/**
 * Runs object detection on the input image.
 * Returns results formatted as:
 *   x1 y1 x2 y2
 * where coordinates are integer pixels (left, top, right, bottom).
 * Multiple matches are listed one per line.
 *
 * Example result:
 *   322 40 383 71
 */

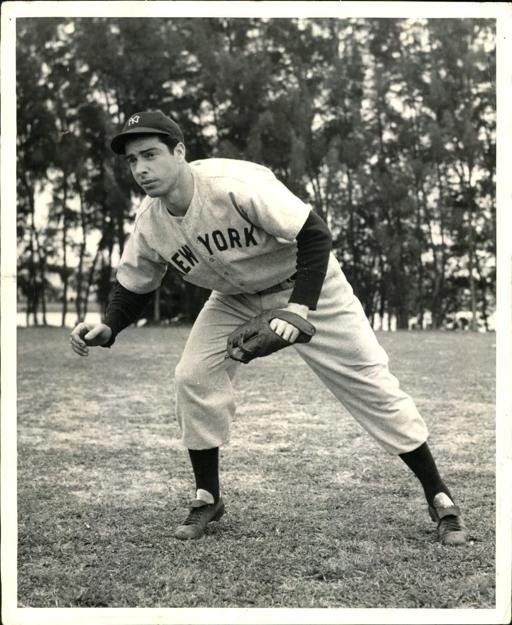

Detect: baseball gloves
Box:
225 310 316 364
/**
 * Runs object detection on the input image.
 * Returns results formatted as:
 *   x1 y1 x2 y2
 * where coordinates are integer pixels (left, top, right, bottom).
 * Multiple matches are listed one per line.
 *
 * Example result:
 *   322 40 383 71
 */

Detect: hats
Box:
110 110 185 156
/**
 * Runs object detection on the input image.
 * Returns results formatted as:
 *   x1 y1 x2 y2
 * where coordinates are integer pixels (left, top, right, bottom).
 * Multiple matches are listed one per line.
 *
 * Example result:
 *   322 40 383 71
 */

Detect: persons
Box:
69 102 468 548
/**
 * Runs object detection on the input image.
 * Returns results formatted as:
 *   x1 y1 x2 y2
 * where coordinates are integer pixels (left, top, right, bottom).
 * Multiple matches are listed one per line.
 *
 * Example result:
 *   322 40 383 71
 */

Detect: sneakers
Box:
428 503 470 545
173 495 226 540
